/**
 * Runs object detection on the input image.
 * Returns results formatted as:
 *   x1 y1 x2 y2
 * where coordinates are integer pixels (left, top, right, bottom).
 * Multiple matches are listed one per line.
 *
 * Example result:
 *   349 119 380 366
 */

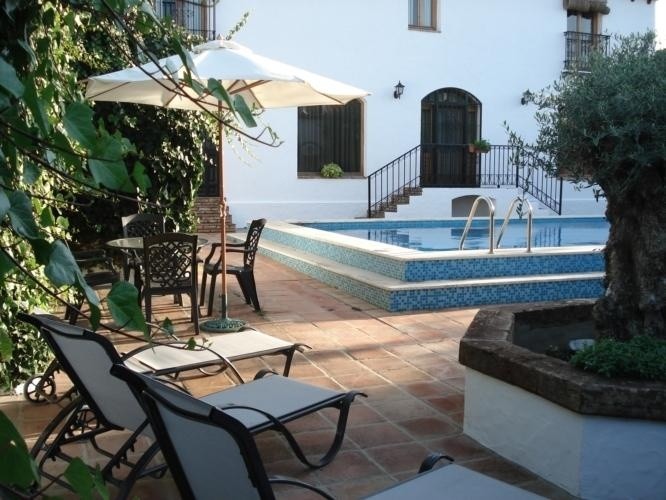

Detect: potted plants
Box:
467 138 492 155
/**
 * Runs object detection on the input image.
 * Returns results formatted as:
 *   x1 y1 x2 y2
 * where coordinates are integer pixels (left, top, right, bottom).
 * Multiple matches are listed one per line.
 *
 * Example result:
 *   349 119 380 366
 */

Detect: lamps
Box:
394 80 405 99
519 88 533 106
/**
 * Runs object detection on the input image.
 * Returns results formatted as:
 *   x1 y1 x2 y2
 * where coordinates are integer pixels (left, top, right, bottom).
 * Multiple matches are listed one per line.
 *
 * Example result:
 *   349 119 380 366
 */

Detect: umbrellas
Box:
77 33 372 318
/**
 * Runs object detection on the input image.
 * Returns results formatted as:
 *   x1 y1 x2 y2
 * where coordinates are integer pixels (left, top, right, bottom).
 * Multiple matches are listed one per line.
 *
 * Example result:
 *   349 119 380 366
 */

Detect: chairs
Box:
23 326 312 439
109 361 551 499
16 313 369 498
60 212 267 326
